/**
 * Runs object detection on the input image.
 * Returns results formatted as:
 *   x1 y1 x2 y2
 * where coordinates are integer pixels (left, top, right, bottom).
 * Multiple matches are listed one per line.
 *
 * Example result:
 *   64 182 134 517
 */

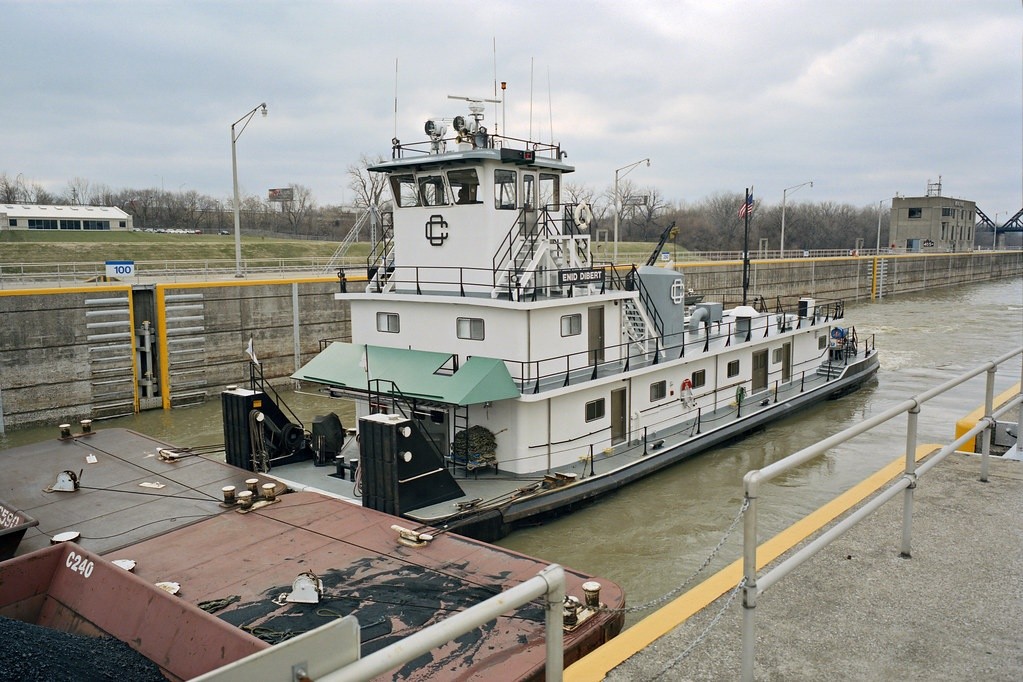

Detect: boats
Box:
222 37 880 543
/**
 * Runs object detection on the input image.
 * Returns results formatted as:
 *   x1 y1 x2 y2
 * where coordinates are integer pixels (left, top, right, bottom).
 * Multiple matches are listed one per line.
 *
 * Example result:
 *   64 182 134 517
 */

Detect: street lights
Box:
780 181 813 259
613 158 650 266
993 211 1007 252
231 102 268 278
877 195 905 256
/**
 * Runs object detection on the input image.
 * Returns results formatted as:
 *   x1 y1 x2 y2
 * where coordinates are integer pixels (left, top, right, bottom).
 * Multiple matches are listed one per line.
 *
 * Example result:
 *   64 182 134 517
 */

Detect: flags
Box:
738 192 753 219
246 338 258 365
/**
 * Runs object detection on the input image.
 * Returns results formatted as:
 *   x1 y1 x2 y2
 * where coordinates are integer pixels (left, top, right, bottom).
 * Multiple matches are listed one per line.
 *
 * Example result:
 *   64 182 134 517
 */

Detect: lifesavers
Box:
682 378 693 403
574 203 591 230
